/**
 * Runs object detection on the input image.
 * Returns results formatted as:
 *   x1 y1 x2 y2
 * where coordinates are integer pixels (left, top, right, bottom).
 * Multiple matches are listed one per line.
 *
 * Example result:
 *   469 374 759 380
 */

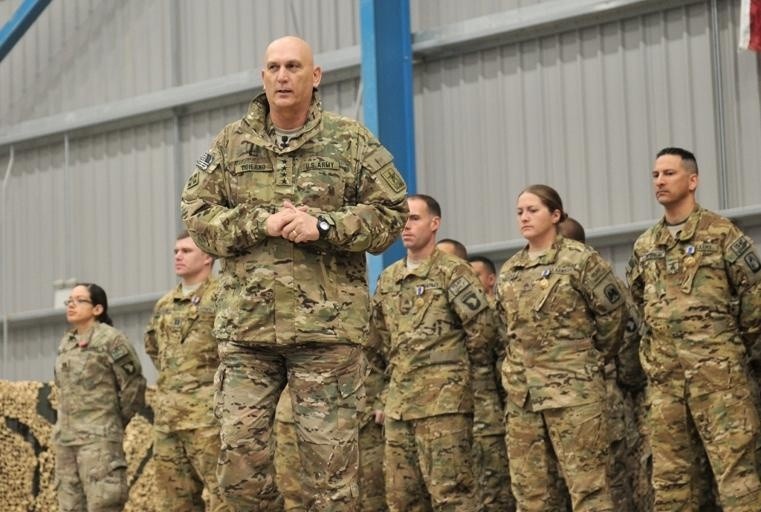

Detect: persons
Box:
51 228 309 511
181 36 410 512
356 147 760 512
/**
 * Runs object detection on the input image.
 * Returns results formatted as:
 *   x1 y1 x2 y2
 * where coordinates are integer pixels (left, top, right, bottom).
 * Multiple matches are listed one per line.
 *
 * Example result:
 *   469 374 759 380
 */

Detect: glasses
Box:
64 297 94 305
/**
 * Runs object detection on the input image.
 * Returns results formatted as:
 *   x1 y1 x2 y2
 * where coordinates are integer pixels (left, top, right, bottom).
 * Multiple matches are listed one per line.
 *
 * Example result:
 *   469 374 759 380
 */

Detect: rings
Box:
293 230 298 238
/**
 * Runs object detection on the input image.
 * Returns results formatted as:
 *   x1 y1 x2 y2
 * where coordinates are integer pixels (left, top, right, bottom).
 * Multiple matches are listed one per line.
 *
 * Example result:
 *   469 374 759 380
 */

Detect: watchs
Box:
317 217 331 240
